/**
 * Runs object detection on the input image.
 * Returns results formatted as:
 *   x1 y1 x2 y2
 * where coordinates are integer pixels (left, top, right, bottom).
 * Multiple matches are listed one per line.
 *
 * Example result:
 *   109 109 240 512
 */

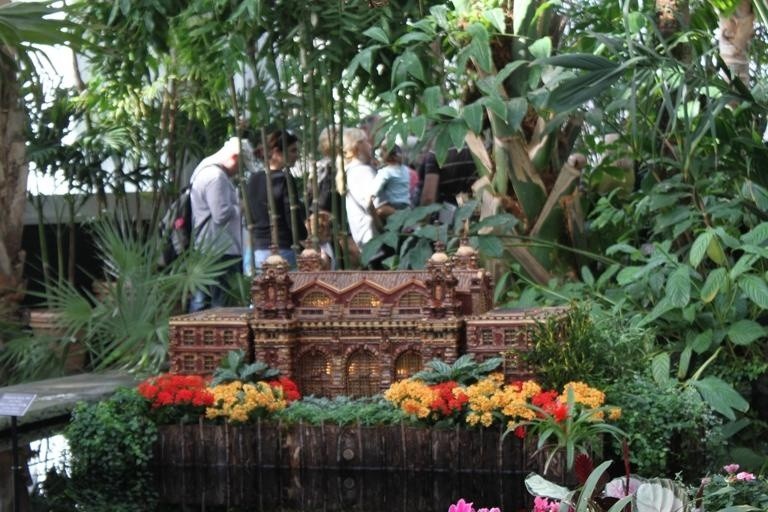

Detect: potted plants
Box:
22 78 165 373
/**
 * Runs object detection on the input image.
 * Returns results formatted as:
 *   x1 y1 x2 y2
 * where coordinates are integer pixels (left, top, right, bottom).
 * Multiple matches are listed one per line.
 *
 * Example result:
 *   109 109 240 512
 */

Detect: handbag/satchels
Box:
366 196 386 233
318 164 340 217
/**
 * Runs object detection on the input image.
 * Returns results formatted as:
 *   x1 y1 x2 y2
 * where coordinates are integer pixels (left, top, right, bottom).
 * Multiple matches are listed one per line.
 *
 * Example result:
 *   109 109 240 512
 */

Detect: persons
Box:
189 84 493 312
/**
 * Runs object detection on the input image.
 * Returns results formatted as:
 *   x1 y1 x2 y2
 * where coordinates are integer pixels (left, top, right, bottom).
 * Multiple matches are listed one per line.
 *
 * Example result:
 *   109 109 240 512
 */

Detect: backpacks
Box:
155 163 222 273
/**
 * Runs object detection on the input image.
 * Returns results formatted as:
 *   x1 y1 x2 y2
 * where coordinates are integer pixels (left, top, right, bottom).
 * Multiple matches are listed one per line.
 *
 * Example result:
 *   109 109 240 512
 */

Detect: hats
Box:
266 130 297 148
230 137 256 174
